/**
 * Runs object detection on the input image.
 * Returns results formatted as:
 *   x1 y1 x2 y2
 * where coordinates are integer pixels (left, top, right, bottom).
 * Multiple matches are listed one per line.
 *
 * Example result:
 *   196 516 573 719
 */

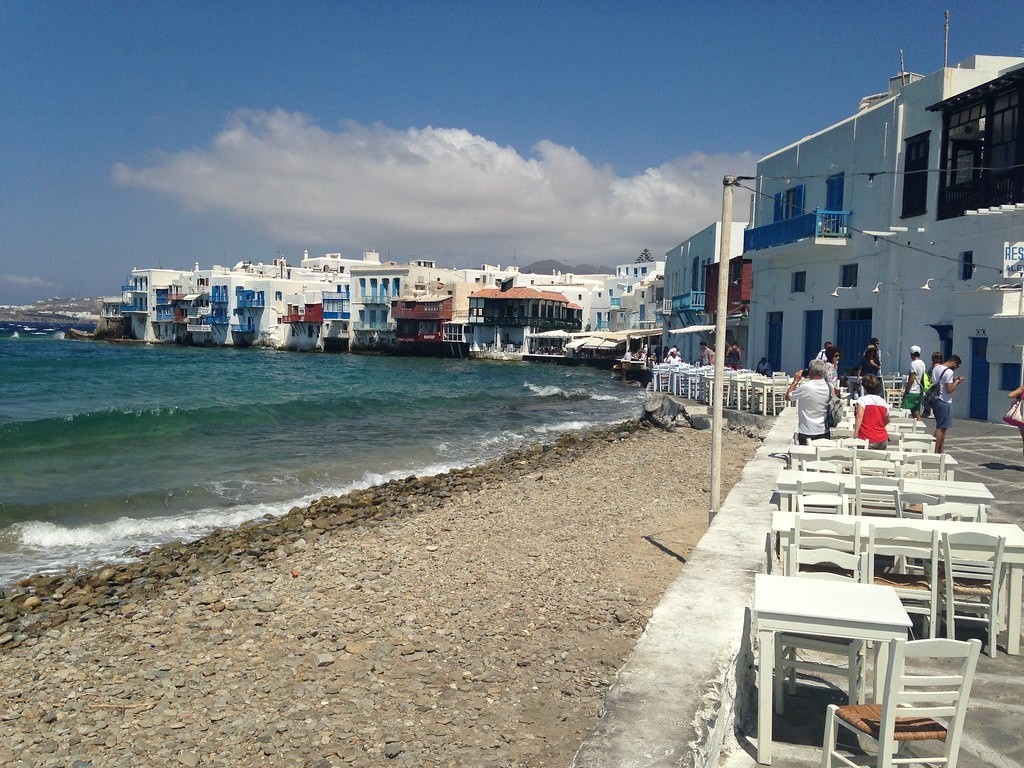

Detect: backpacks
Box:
825 383 843 427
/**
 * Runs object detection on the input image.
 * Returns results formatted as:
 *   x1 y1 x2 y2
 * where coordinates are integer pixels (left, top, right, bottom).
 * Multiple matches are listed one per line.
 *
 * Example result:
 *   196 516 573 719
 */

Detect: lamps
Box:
830 287 852 297
872 282 882 292
919 278 935 291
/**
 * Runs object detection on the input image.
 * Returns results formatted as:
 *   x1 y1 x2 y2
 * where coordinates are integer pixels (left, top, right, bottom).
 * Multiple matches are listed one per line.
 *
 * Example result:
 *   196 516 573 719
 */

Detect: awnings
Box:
668 325 716 334
598 339 619 349
583 337 605 349
564 339 587 348
183 294 201 300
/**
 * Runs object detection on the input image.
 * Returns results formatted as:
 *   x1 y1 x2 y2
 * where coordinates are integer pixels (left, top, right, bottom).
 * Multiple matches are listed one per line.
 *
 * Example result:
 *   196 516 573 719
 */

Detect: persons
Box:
853 373 890 450
923 352 963 454
815 342 832 362
531 347 555 355
757 357 772 377
856 338 884 397
822 346 840 385
662 345 684 363
901 344 926 421
697 341 716 366
1008 387 1024 445
726 340 743 370
622 346 662 365
785 360 835 445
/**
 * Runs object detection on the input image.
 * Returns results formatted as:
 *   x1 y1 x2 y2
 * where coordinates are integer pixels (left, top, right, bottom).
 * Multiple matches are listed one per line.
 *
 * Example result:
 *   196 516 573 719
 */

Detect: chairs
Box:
651 361 1006 768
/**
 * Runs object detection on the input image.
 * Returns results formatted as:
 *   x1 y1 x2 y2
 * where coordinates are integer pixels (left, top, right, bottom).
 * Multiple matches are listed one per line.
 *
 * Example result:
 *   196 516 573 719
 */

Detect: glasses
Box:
835 355 840 358
875 343 879 345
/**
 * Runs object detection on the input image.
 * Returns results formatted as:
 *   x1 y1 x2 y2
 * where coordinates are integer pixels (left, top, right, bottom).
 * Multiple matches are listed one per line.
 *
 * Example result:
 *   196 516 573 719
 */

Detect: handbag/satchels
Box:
1003 399 1024 426
922 383 940 406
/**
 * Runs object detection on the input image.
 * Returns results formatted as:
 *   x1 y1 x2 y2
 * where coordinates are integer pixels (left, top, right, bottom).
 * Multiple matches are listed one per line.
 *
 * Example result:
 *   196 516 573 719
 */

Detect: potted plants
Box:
846 367 865 399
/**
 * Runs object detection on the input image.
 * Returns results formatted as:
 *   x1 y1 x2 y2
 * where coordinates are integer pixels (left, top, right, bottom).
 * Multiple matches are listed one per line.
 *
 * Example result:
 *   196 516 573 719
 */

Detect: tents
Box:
525 329 572 355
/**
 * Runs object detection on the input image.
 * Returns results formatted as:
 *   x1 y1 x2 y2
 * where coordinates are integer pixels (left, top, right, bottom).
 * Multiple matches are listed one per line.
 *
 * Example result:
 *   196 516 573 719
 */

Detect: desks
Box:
788 444 958 482
652 366 731 392
830 420 927 433
841 407 912 418
670 369 754 396
729 378 804 411
683 373 762 400
700 376 772 407
752 572 913 766
884 380 922 416
776 470 994 523
750 380 809 417
770 511 1024 655
794 433 938 445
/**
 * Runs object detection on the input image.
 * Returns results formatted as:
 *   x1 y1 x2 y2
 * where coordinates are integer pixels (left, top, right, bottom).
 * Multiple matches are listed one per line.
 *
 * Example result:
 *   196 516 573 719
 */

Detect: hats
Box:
910 345 921 354
